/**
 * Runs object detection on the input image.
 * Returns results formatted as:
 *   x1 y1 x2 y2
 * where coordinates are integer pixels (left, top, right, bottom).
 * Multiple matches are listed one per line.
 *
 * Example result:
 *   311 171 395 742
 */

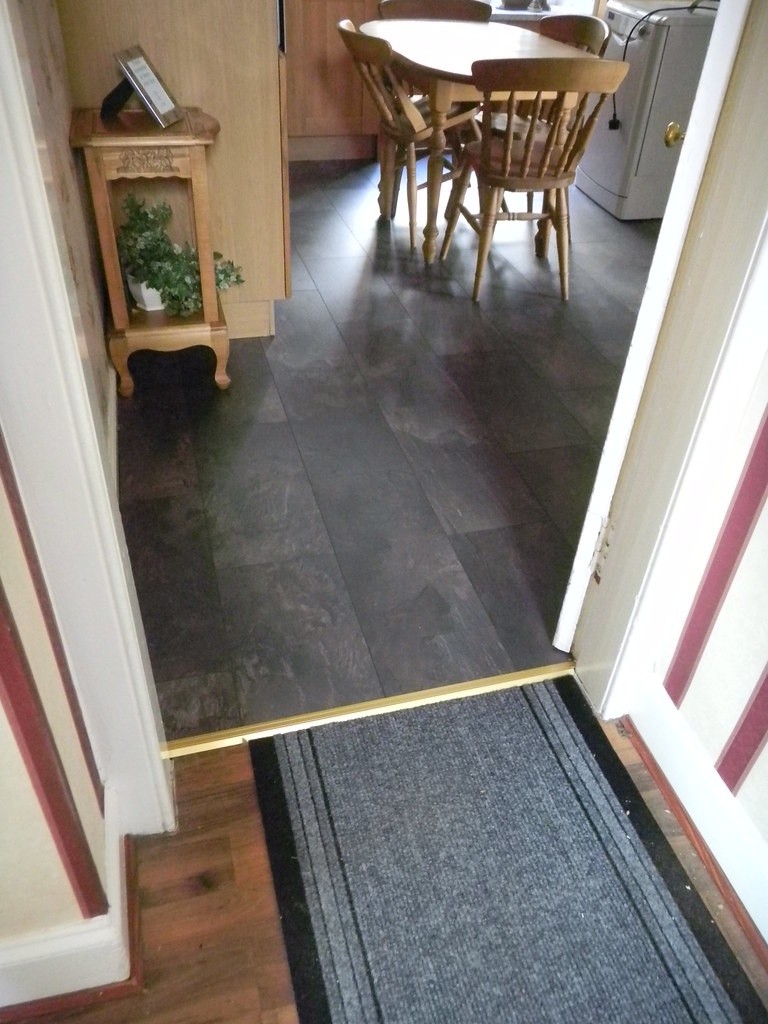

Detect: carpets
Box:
249 675 768 1024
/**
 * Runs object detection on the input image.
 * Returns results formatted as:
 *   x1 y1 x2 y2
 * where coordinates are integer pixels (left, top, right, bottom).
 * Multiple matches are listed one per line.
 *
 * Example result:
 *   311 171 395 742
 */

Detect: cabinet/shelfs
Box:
57 0 293 338
70 106 230 396
284 0 491 161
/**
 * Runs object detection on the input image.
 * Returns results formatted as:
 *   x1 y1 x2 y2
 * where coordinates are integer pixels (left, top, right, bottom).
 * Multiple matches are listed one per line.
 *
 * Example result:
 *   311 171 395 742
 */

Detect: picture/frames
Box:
114 43 186 129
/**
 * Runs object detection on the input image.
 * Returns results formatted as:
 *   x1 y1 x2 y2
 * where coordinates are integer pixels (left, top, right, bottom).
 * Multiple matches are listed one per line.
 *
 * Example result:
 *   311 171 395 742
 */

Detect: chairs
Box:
442 14 611 256
439 61 630 302
375 0 490 218
337 17 510 248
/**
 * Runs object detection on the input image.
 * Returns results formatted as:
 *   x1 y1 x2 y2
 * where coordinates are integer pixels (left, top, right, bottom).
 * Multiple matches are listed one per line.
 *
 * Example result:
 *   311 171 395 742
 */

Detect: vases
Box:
126 265 168 310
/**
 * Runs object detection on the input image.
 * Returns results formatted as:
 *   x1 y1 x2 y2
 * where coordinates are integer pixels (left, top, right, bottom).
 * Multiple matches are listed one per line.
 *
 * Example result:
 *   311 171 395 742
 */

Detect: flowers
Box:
117 196 243 318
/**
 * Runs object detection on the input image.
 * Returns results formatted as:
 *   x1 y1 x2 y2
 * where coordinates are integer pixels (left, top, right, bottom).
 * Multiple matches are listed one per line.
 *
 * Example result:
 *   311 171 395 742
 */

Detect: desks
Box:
361 18 600 258
